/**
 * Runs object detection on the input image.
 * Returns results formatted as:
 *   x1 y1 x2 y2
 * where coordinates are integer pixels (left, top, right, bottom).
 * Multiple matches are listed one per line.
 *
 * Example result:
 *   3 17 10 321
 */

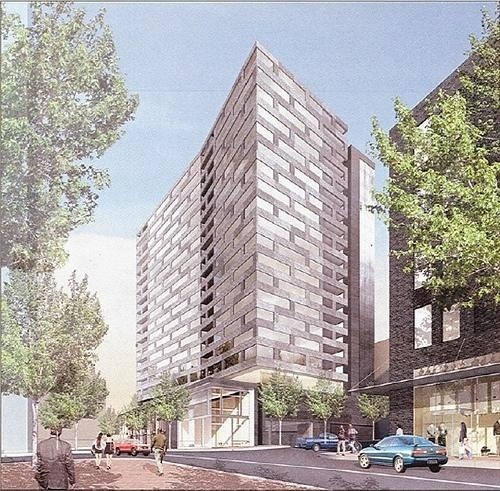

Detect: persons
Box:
336 425 345 456
151 427 168 477
493 418 500 457
347 424 359 454
35 426 76 491
426 423 435 444
104 433 116 471
437 422 448 447
458 422 472 459
395 424 403 436
91 432 106 469
